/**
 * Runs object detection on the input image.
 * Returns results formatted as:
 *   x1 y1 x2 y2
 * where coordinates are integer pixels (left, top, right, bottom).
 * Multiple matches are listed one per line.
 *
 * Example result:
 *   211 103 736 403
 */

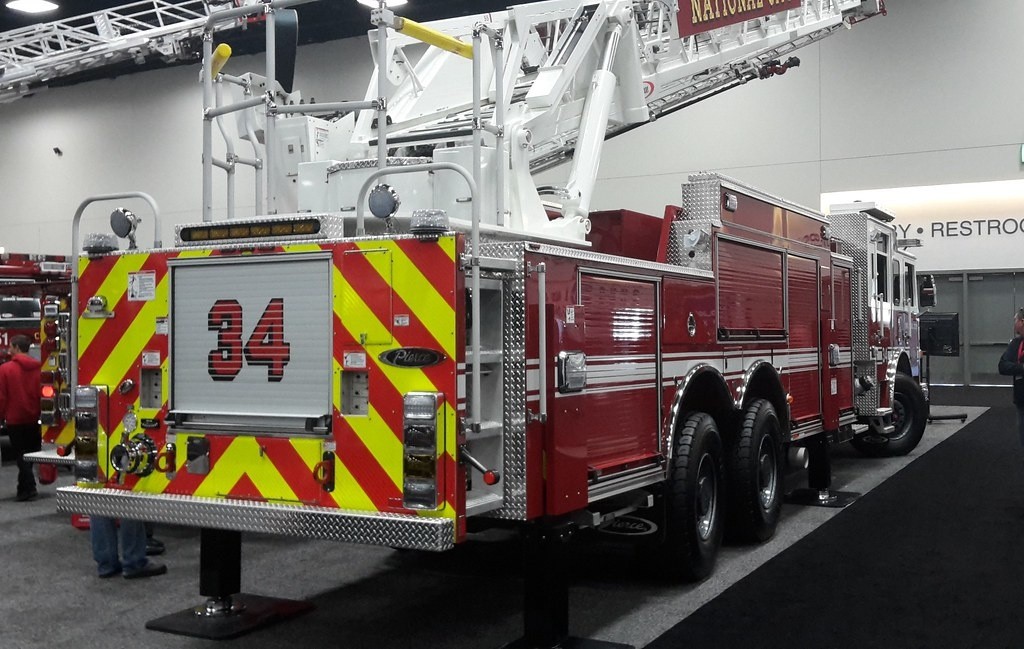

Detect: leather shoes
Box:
149 538 164 547
145 545 164 555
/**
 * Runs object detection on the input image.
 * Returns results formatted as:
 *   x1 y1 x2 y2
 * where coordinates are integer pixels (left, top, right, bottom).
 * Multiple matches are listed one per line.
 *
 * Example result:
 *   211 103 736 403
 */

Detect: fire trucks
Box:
0 0 937 604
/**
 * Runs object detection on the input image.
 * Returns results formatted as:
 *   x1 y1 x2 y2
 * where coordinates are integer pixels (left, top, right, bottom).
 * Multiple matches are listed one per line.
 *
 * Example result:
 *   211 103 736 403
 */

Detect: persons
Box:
998 308 1024 450
89 514 166 579
0 335 42 503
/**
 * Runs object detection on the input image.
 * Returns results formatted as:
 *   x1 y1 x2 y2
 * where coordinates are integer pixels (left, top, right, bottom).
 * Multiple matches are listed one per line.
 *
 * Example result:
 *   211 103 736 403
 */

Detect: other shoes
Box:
15 486 38 500
123 563 167 578
99 561 124 579
